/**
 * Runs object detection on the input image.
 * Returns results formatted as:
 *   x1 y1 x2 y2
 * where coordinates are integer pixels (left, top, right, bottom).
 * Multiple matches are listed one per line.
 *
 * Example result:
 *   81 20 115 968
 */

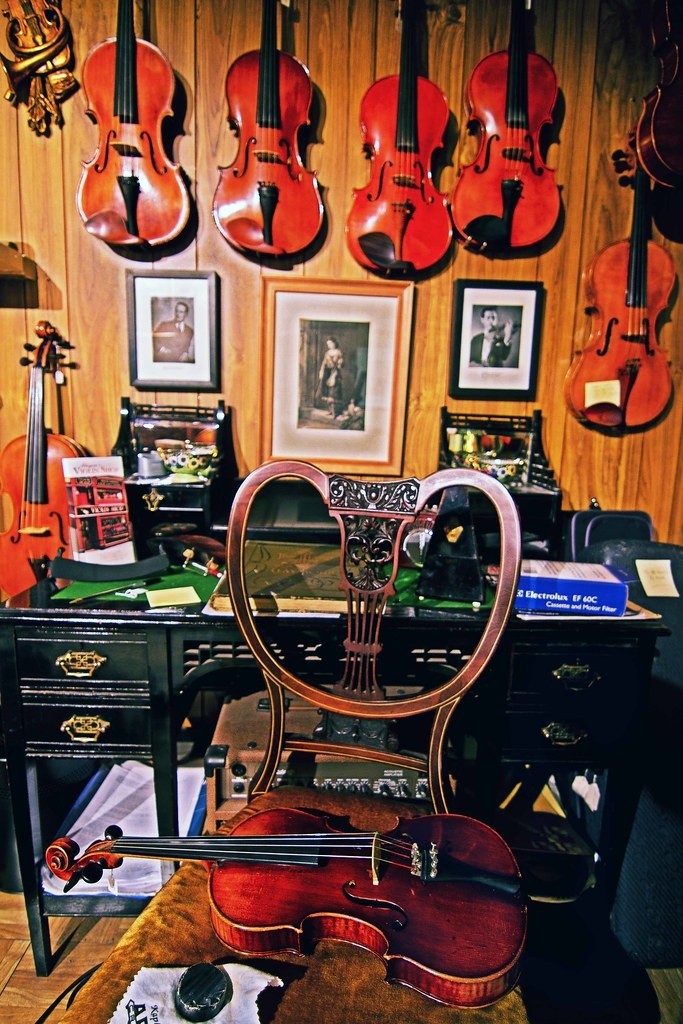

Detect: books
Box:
211 540 396 617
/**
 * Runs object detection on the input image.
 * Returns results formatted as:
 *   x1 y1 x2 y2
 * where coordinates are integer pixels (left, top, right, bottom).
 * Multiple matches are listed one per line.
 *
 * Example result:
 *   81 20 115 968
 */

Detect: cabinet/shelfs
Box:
112 398 563 565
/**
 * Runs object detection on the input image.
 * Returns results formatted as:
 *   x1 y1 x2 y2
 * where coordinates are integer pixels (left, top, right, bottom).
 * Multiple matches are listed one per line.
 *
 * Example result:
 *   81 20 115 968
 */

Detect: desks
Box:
0 540 674 978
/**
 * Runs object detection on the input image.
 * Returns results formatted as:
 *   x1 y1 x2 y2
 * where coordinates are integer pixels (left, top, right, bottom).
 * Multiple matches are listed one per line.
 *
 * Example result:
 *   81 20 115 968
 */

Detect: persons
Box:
470 305 515 368
154 302 194 361
315 337 344 415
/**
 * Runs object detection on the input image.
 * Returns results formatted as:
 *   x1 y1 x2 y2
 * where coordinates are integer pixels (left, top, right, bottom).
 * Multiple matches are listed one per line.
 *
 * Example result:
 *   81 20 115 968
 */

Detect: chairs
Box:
56 458 533 1024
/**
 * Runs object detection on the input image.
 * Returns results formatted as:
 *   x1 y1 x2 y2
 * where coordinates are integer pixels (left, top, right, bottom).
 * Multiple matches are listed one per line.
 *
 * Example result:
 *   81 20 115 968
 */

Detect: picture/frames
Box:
448 278 544 400
125 269 220 394
259 277 414 477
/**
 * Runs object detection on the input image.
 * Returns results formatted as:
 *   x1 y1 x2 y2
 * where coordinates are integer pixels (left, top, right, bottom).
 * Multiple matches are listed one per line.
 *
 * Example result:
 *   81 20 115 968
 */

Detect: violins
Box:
449 0 561 254
74 0 192 249
44 804 531 1010
211 0 325 261
344 0 455 279
635 0 683 188
0 320 90 599
562 122 679 432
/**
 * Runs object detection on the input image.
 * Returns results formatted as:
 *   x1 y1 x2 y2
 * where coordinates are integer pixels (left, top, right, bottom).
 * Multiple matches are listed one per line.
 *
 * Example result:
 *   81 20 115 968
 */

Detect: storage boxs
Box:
203 684 435 831
512 559 641 617
